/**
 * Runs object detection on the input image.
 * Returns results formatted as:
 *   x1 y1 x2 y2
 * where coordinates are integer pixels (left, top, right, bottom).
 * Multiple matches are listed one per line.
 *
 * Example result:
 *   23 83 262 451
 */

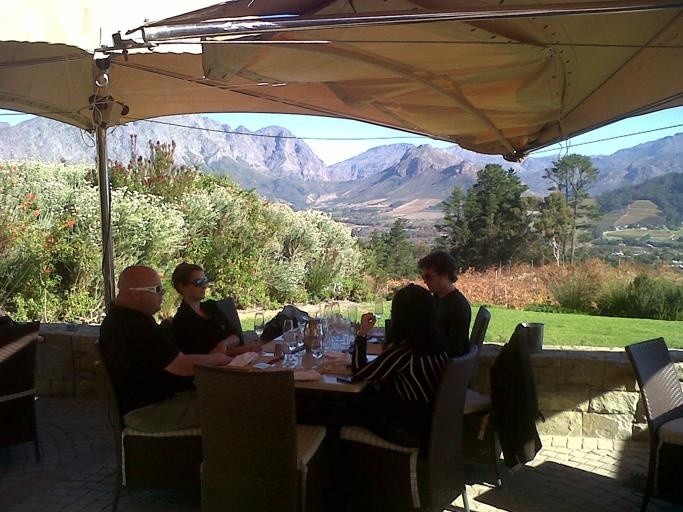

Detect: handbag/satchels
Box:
260 305 311 340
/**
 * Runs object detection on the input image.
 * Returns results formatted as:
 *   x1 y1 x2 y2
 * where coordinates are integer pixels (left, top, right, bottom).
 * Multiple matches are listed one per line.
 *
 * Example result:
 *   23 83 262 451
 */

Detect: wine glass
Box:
373 297 383 333
281 301 358 368
252 311 265 342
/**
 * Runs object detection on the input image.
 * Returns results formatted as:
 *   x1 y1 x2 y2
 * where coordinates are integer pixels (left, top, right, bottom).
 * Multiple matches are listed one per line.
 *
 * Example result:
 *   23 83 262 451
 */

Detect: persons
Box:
417 250 472 358
169 262 264 371
320 284 452 503
0 329 44 363
99 266 209 430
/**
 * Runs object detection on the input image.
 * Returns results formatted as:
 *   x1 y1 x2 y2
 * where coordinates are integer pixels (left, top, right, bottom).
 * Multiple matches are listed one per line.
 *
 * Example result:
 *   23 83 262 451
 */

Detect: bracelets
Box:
354 328 368 339
28 332 37 343
218 342 227 348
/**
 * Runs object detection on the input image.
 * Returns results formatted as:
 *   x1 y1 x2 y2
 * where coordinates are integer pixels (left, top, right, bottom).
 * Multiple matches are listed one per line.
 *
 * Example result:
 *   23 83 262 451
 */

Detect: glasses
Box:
189 278 209 288
421 272 438 283
129 285 163 294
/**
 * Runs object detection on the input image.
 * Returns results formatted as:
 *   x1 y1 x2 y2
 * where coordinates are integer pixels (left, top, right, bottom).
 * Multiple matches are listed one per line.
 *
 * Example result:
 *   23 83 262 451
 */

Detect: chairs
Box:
623 335 682 511
0 315 40 462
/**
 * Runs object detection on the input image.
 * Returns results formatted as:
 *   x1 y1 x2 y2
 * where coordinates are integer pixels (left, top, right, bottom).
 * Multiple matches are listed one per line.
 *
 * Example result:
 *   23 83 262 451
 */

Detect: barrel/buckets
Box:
523 323 545 354
